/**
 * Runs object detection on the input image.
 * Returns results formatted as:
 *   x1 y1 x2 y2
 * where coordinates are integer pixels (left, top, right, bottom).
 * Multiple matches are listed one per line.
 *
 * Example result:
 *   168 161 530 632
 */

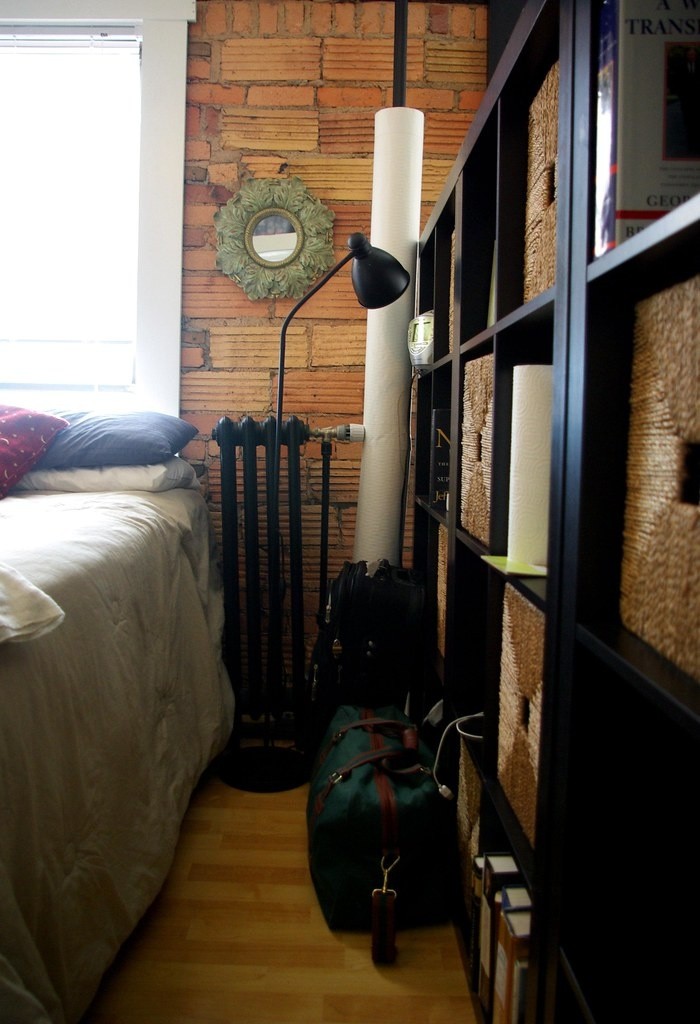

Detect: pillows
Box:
0 404 71 501
10 455 202 492
32 410 200 470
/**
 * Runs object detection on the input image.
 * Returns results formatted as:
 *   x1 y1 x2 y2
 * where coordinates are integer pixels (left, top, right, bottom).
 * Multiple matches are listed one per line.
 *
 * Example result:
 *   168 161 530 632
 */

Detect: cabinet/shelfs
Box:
394 0 700 1024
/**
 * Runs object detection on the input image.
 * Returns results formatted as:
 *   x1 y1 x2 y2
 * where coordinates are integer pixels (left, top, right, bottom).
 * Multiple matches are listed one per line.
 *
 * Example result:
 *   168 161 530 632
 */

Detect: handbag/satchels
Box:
306 704 452 967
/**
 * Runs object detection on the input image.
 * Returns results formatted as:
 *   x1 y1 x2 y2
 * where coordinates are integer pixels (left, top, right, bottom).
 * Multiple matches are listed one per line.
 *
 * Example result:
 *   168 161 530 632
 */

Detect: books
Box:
466 853 532 1024
593 0 700 260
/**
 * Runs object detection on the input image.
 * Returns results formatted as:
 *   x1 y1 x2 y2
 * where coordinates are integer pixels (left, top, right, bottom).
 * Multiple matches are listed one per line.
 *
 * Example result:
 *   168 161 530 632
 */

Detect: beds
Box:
0 486 238 1024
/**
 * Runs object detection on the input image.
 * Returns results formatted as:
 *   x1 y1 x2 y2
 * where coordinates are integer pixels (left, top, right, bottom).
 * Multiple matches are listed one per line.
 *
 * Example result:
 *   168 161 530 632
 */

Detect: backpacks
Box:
301 558 423 755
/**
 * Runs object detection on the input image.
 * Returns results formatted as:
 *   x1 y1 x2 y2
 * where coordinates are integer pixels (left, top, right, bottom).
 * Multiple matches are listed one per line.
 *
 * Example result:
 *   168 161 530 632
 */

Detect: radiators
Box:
212 415 363 749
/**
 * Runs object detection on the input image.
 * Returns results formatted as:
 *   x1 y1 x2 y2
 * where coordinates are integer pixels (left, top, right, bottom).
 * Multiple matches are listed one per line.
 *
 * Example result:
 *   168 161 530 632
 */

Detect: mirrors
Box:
216 175 337 300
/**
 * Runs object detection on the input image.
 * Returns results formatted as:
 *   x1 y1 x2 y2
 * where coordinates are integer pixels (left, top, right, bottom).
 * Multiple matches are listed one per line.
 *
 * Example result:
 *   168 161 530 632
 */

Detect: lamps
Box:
215 233 412 794
